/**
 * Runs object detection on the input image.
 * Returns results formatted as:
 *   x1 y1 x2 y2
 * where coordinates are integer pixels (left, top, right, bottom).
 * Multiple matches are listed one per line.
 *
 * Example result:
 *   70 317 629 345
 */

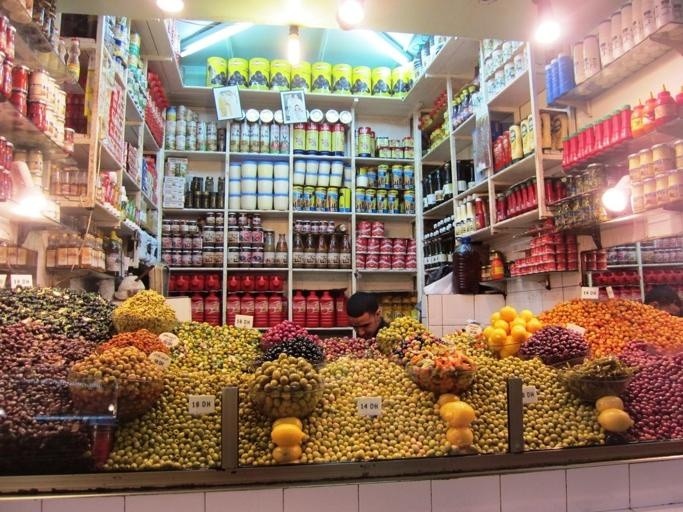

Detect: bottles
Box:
546 64 556 105
293 220 352 269
453 237 482 295
320 291 335 328
352 66 372 94
482 39 529 98
550 59 560 98
165 105 226 153
0 0 81 202
554 163 625 221
292 290 306 328
147 73 169 149
422 159 475 211
493 106 574 172
562 84 683 169
455 193 490 235
331 63 353 94
628 140 683 212
372 67 392 95
271 59 291 90
581 238 683 285
226 57 248 89
452 81 481 129
228 160 289 210
248 57 270 89
355 164 415 214
230 107 289 154
293 159 351 212
417 89 450 156
335 292 348 327
558 51 575 95
126 32 148 119
185 177 225 209
574 0 675 85
207 56 226 87
45 231 122 278
391 67 413 98
312 61 332 93
227 212 288 268
168 274 221 326
226 274 288 328
423 214 455 269
290 60 311 91
162 212 223 267
306 291 320 327
51 169 140 226
496 174 567 222
293 108 352 157
354 127 415 159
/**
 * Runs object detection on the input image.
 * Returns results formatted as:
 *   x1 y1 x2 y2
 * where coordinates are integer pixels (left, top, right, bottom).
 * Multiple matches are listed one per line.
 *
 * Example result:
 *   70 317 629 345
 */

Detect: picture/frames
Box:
213 87 243 121
281 92 309 125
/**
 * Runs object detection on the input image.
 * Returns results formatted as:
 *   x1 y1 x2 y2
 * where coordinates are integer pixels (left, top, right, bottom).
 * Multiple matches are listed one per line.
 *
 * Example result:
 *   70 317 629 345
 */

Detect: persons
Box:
643 286 682 317
347 292 391 339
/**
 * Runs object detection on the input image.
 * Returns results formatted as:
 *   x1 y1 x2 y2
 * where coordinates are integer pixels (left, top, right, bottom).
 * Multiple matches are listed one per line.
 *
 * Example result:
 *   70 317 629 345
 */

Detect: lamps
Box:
284 31 308 74
535 18 562 45
336 0 366 28
156 1 185 14
12 161 49 214
602 175 633 215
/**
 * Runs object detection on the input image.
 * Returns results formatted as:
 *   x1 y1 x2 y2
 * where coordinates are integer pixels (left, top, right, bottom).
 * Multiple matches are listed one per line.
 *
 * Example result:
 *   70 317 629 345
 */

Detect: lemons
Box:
445 426 473 448
594 395 624 411
271 416 303 431
596 409 634 433
271 446 302 463
438 393 459 405
482 305 541 358
270 424 310 446
438 400 475 426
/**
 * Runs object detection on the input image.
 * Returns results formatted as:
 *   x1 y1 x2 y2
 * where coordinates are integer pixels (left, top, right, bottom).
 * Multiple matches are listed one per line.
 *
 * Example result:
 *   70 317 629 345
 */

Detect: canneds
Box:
480 217 578 282
356 221 417 268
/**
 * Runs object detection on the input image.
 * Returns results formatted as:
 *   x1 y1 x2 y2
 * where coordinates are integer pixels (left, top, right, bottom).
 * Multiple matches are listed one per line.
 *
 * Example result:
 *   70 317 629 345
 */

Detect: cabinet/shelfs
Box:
161 88 427 341
405 1 683 281
5 1 185 294
